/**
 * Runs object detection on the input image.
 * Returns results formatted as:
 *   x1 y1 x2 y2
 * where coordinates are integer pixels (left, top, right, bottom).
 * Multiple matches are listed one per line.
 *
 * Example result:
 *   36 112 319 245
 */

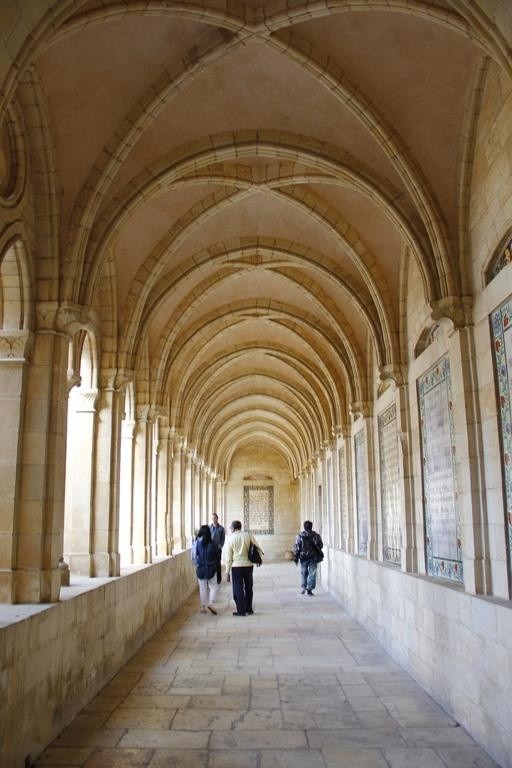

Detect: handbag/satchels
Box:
248 535 264 568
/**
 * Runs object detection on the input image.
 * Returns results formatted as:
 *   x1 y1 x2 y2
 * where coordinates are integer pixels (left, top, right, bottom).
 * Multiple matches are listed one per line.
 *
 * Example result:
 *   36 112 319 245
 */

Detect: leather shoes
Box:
233 610 253 616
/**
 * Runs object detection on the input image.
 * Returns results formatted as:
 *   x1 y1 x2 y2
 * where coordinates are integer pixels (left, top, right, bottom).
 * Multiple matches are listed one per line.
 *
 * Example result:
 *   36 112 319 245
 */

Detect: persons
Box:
294 520 323 596
190 525 222 615
223 520 263 617
205 513 225 584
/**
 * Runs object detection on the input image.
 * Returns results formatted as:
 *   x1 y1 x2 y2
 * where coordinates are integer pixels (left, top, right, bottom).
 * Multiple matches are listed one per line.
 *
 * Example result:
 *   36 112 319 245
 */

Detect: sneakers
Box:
199 606 218 615
301 588 314 596
